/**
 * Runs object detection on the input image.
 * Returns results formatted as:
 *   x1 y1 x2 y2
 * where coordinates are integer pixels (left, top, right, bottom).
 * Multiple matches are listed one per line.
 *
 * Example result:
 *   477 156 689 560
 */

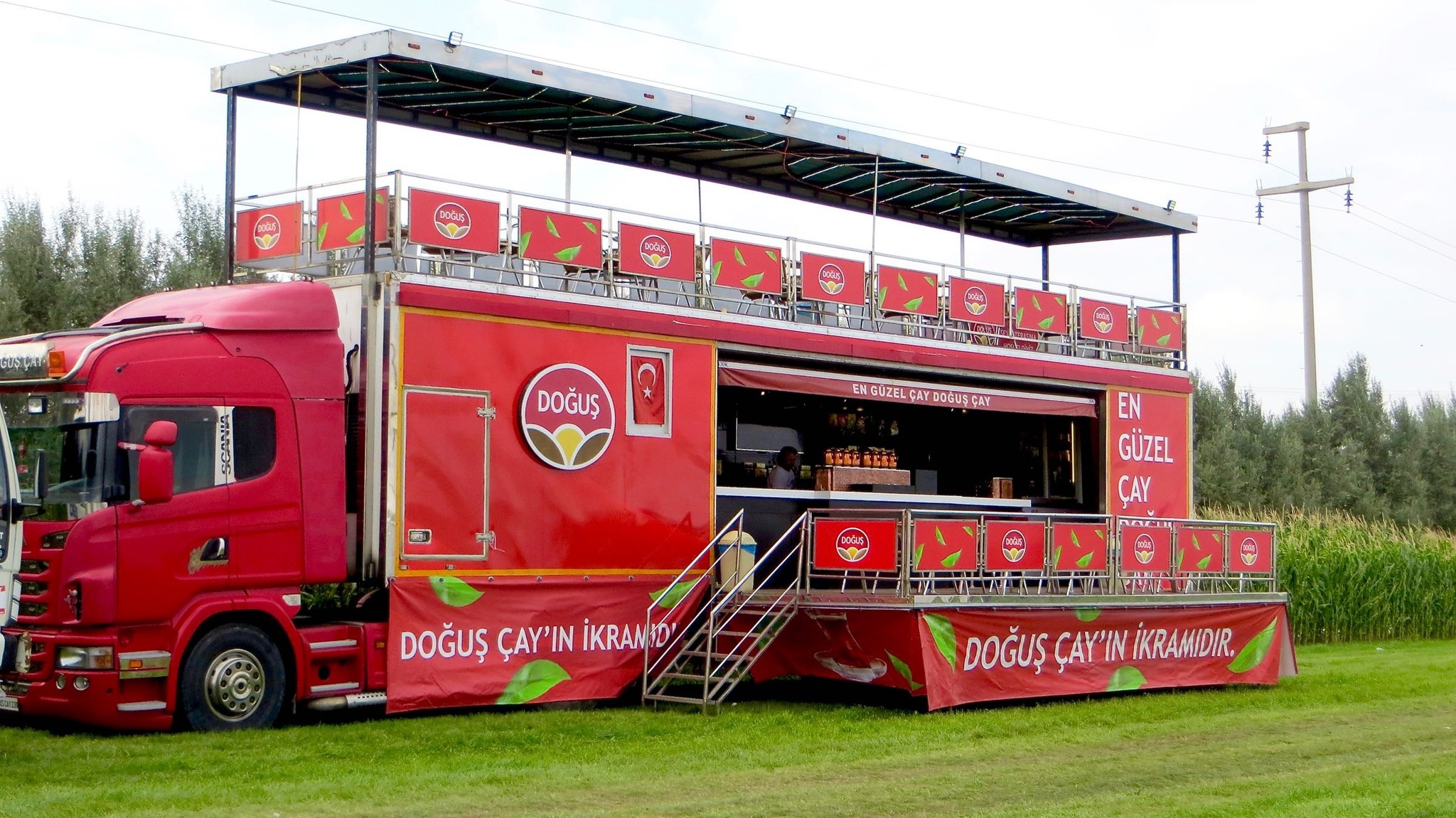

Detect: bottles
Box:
800 465 811 480
755 463 767 478
1029 481 1035 497
814 465 828 491
826 449 897 468
744 462 753 474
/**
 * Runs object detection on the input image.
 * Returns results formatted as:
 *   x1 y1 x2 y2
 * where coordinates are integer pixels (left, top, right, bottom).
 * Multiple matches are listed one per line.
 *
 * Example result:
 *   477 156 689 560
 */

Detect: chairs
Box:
344 195 1154 366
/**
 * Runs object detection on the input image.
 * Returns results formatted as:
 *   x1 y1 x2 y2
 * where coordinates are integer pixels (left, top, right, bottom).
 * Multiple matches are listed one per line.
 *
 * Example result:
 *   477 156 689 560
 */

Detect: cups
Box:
992 477 1013 499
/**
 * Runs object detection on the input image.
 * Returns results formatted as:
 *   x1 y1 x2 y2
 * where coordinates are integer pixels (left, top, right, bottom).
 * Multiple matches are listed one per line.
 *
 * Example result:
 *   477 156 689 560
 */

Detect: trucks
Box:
2 30 1191 732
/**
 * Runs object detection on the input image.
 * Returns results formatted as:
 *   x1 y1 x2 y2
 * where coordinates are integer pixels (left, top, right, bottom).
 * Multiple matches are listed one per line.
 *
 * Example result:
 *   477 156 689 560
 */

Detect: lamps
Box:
784 106 797 124
956 146 967 164
445 31 463 53
1167 200 1176 214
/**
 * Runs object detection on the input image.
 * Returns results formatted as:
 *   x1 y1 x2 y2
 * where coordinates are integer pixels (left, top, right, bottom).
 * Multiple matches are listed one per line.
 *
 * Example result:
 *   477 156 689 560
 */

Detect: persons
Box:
762 446 799 549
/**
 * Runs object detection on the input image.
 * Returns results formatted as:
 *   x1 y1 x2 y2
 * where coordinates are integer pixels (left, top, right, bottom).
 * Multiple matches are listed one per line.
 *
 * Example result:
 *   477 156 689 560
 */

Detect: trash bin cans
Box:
717 531 758 593
